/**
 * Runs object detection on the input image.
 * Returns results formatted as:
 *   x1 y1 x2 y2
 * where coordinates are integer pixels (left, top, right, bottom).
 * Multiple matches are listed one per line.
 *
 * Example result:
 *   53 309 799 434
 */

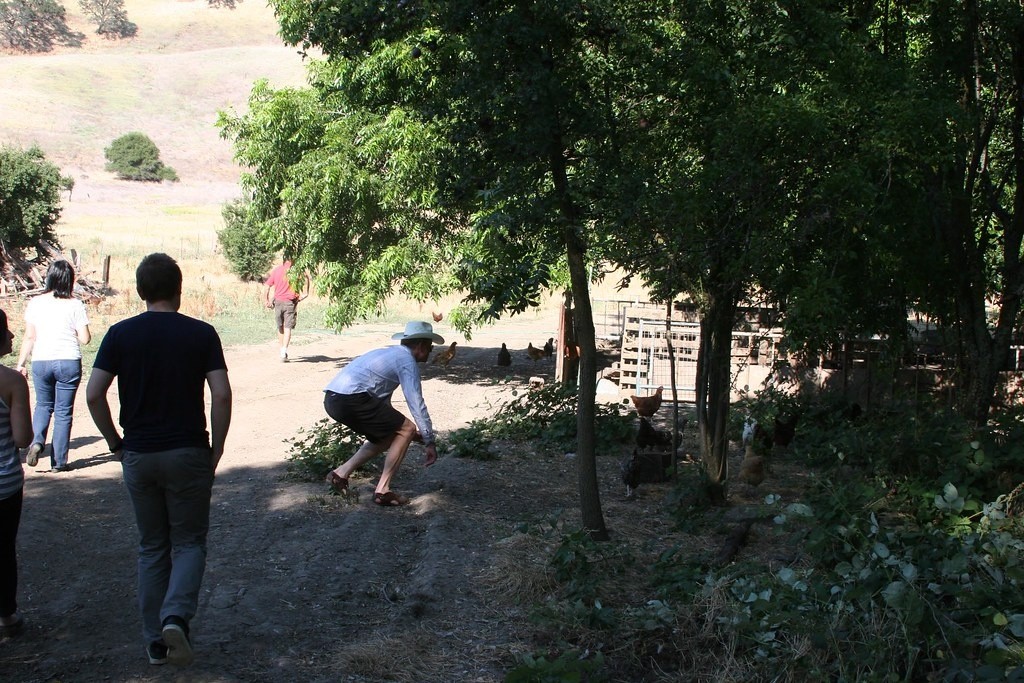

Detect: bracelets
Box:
109 438 122 453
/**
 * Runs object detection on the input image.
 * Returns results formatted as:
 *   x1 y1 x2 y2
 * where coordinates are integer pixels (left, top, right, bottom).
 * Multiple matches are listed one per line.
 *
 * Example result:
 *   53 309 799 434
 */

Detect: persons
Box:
17 260 91 473
264 246 310 362
0 309 35 634
324 323 438 505
86 253 232 667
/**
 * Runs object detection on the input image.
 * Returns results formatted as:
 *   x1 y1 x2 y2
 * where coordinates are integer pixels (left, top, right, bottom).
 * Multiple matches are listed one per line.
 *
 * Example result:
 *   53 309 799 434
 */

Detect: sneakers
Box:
26 442 43 466
53 464 73 472
161 614 195 666
0 614 23 636
282 357 289 362
146 641 168 663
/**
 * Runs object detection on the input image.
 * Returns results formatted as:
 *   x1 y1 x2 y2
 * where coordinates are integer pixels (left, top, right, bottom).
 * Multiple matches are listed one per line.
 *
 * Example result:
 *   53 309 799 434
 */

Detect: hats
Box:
391 320 445 344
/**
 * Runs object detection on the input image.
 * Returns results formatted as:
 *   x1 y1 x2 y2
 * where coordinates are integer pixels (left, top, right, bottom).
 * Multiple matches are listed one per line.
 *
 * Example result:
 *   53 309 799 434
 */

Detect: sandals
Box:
372 491 409 505
326 470 359 501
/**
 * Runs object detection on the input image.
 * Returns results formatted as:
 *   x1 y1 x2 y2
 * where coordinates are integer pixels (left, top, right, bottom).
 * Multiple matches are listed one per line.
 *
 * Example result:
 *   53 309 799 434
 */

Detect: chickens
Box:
772 416 797 450
430 341 458 372
738 445 765 497
620 445 646 497
635 418 657 453
527 342 547 365
664 418 689 452
432 311 443 323
544 337 554 362
497 343 512 373
630 386 665 422
